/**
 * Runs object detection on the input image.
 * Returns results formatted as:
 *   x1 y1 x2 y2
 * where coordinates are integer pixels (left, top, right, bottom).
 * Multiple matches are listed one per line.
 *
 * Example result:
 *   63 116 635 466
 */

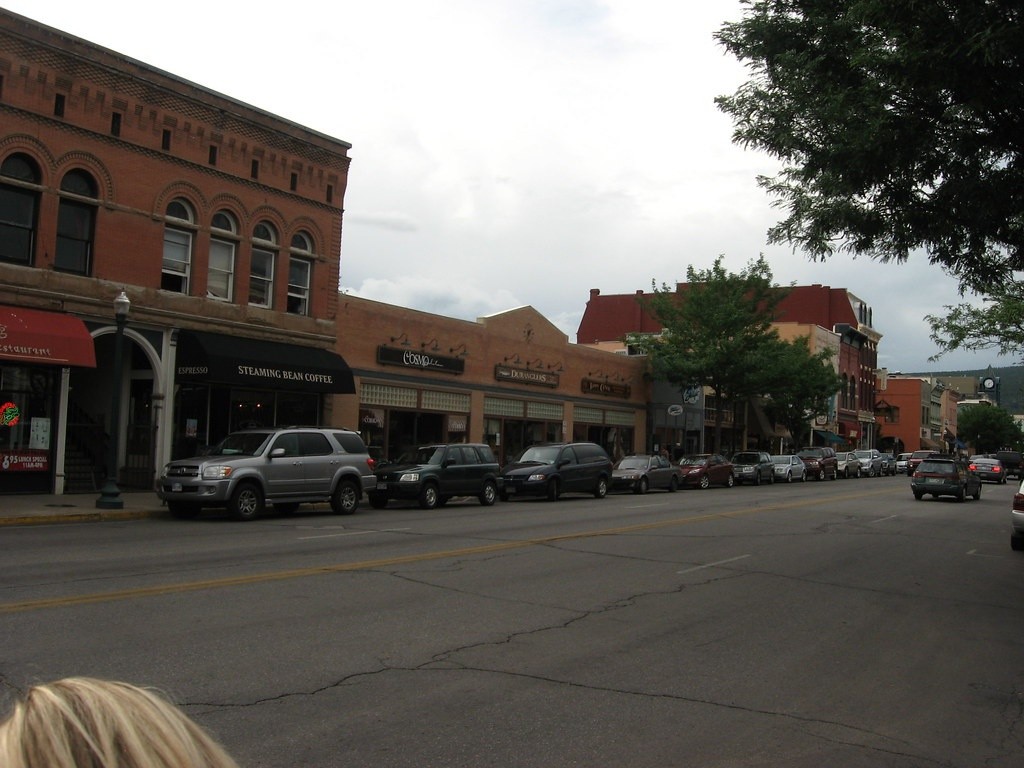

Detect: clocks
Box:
984 379 994 389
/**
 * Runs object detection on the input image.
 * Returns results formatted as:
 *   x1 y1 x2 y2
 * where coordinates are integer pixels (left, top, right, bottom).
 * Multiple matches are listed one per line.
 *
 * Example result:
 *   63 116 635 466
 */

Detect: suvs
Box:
732 451 776 486
155 426 380 523
795 447 838 482
497 441 616 501
369 444 505 509
854 448 883 478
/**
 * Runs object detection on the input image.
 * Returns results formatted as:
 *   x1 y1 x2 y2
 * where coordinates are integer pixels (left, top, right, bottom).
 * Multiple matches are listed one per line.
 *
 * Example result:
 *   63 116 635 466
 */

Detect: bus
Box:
909 455 982 502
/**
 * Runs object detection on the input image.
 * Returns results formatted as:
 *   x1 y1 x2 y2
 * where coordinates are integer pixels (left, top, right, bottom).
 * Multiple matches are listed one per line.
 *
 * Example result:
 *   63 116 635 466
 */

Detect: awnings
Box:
175 329 356 394
0 304 96 368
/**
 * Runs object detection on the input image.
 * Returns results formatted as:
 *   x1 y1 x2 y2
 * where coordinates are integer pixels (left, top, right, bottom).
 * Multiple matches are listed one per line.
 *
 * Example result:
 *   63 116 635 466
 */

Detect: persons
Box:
0 675 239 768
659 447 669 460
673 441 685 461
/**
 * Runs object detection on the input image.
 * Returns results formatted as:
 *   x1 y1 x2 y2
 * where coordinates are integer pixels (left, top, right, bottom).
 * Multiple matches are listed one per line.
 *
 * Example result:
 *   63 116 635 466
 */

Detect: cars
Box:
896 453 913 474
772 454 807 483
1011 478 1024 551
679 453 735 489
970 459 1007 484
880 453 897 476
835 451 861 479
611 455 683 493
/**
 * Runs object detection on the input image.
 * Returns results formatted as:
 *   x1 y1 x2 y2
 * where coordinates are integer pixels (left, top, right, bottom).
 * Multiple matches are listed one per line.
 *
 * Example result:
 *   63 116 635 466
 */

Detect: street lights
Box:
95 292 131 508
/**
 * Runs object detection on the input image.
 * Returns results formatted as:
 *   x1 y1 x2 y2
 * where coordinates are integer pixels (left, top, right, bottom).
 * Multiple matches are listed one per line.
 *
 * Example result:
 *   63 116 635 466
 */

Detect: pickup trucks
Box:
996 451 1024 481
908 450 941 476
